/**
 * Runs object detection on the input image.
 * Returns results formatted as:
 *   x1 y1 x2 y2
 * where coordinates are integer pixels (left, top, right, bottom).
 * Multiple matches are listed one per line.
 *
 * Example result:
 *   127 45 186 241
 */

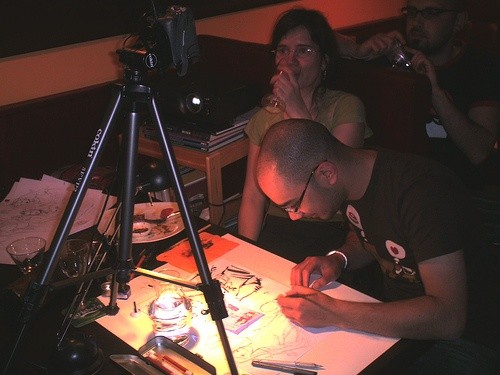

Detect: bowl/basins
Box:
132 222 151 238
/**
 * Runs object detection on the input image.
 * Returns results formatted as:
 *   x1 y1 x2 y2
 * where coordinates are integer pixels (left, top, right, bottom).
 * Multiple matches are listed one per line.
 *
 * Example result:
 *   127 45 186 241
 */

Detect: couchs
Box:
0 35 426 262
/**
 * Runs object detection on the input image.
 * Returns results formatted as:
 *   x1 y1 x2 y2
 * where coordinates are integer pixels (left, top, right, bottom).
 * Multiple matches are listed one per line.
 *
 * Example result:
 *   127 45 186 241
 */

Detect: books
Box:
140 111 248 153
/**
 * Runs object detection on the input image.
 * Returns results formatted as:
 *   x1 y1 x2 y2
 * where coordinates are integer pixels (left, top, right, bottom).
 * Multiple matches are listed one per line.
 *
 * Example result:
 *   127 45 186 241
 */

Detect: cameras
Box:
387 45 413 70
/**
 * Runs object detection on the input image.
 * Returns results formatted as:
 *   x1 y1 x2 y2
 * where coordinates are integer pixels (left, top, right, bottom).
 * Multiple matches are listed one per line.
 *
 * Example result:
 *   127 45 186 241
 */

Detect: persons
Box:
238 8 363 265
341 0 500 185
256 118 500 375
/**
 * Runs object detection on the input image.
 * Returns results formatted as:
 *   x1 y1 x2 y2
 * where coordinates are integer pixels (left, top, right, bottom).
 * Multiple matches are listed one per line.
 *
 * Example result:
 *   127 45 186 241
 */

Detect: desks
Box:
0 133 419 375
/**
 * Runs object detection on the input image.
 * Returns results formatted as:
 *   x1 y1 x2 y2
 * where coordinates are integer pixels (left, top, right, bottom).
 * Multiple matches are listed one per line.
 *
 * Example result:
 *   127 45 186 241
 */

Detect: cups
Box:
6 237 47 279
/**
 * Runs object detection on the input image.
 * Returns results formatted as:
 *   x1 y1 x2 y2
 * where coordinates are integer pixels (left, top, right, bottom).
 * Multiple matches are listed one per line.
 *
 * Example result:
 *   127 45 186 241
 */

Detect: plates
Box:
96 201 184 244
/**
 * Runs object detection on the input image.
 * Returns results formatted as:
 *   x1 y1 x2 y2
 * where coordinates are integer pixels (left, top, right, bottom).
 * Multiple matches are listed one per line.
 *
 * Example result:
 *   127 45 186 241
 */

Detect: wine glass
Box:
57 239 101 317
260 57 301 114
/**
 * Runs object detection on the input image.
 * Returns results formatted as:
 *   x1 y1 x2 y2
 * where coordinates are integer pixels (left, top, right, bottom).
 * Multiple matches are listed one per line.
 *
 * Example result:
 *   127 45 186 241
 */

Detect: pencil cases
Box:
109 336 216 375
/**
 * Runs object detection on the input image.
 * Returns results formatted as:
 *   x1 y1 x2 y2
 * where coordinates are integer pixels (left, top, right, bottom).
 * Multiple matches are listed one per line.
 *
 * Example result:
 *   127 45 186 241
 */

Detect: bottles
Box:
147 269 192 348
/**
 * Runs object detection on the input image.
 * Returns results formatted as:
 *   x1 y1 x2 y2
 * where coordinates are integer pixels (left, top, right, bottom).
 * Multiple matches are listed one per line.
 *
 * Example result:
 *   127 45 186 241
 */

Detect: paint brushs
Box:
129 255 146 278
167 223 212 251
277 293 317 299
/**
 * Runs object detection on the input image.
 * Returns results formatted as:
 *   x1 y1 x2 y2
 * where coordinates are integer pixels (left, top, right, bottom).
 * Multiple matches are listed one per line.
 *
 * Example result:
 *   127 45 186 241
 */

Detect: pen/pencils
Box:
265 362 323 368
252 360 317 375
155 352 194 375
146 357 173 375
134 301 137 312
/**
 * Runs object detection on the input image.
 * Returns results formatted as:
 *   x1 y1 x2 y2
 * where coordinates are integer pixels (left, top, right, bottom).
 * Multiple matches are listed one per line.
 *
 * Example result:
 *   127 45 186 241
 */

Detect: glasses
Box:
278 159 327 212
401 7 462 20
271 46 323 58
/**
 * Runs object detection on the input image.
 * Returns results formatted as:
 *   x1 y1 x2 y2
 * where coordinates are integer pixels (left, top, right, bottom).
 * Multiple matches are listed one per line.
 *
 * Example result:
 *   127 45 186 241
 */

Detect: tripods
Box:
5 87 239 375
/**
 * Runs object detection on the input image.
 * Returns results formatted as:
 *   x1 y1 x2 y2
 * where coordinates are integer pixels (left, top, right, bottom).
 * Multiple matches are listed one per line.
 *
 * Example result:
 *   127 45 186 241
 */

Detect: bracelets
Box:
327 248 349 272
